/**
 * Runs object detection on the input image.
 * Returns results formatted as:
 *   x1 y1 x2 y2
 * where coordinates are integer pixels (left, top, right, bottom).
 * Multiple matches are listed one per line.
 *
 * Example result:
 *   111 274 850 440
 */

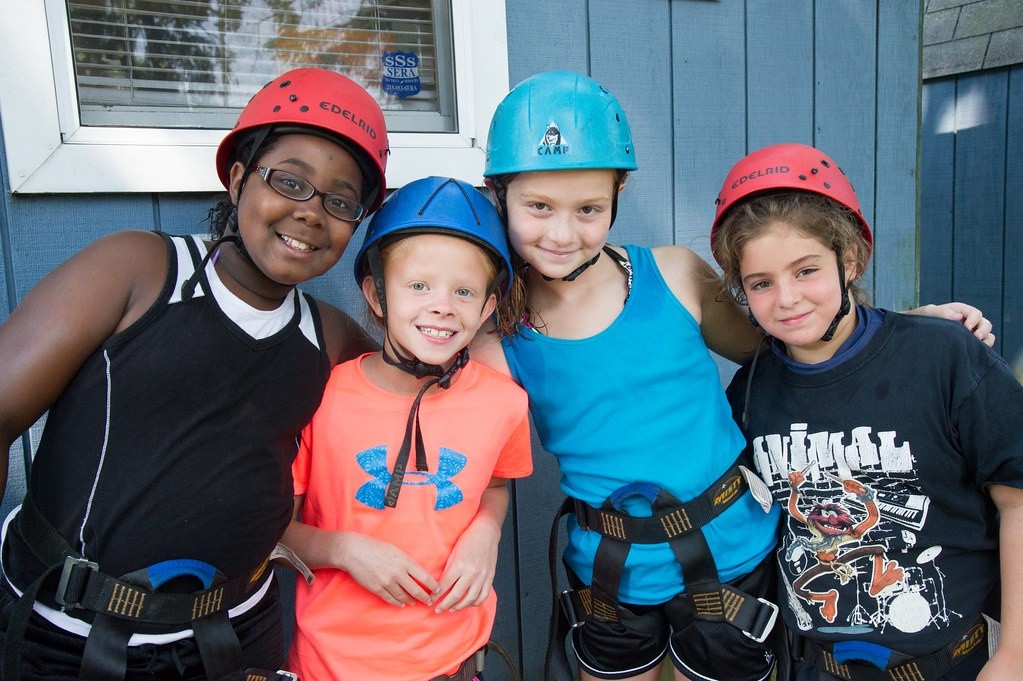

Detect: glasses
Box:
247 163 368 223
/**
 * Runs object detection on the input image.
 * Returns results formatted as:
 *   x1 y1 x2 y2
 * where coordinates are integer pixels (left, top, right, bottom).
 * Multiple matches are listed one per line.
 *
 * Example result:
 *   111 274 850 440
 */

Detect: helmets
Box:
215 68 389 220
482 69 640 178
352 176 515 308
710 144 873 273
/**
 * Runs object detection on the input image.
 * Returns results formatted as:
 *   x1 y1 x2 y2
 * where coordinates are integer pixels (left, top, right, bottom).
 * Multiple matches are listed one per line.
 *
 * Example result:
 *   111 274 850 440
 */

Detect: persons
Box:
2 72 390 681
702 142 1022 681
474 64 998 681
275 172 535 680
